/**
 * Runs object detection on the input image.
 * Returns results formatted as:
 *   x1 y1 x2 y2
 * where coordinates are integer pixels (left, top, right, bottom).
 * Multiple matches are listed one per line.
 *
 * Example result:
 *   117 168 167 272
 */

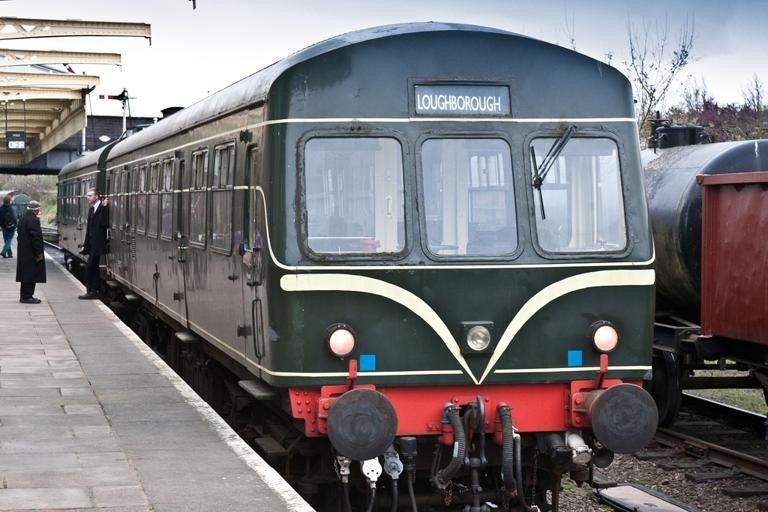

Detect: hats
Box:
27 200 41 210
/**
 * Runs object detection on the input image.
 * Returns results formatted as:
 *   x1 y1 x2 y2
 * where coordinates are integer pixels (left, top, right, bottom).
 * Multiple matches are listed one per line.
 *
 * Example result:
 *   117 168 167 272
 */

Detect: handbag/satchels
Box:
5 221 15 232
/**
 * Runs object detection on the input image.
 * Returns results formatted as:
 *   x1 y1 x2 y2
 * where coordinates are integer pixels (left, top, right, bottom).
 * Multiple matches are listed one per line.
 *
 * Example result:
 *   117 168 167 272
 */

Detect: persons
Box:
0 194 17 259
15 201 47 304
78 187 109 300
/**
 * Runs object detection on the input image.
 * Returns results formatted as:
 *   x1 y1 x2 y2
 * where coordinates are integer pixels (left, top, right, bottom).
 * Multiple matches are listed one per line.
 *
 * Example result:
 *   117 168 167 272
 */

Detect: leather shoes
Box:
20 297 41 303
79 279 106 299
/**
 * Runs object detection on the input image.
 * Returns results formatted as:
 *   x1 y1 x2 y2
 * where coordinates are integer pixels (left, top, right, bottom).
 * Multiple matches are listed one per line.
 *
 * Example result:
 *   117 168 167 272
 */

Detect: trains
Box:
639 112 767 429
55 20 659 511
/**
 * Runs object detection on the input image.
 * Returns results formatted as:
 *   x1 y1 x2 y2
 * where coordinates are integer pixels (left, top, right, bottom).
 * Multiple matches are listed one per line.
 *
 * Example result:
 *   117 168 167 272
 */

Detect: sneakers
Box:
0 252 13 258
